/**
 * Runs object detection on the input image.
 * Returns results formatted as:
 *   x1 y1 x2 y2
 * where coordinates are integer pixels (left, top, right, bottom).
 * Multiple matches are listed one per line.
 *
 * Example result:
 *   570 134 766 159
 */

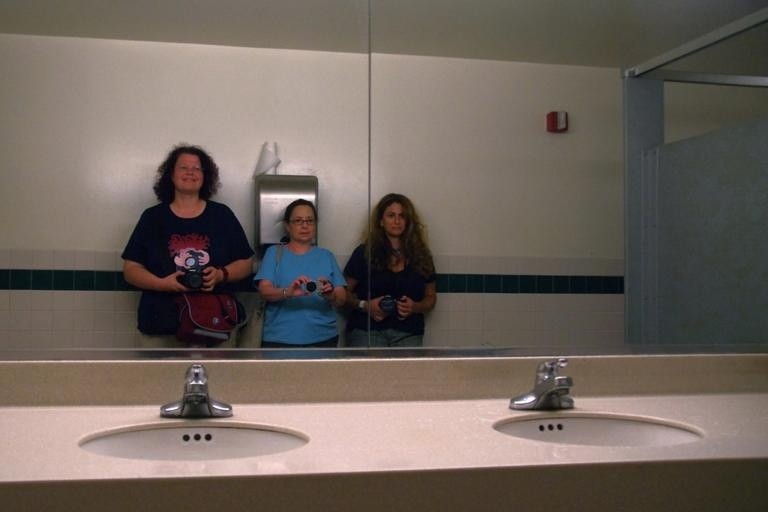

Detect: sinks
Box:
491 413 703 446
78 421 311 459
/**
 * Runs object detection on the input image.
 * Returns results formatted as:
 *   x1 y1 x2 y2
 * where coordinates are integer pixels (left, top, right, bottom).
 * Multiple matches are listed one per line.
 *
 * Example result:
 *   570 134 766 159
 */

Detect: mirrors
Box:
0 0 767 361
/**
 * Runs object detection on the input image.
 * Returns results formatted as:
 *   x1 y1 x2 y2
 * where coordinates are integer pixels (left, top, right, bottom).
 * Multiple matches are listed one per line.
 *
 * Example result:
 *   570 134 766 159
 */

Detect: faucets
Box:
158 364 232 418
507 358 575 413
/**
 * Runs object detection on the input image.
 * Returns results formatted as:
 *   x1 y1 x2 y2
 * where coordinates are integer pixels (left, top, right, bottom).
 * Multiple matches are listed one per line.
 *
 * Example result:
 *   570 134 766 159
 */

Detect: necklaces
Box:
393 247 401 254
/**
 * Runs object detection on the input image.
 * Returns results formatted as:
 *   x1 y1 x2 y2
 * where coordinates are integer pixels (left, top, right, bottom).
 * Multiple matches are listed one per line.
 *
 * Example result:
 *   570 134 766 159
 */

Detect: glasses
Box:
177 166 202 174
287 218 315 226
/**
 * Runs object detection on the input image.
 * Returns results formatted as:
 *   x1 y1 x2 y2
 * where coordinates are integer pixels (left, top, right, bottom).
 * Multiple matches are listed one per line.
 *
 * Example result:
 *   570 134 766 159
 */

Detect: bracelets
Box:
358 299 366 313
283 288 291 300
327 293 337 302
218 267 229 283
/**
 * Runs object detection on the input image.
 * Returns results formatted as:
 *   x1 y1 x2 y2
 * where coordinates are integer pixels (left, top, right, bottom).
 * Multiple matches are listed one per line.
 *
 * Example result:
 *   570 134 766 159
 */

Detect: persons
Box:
121 143 252 349
340 193 436 347
251 200 346 348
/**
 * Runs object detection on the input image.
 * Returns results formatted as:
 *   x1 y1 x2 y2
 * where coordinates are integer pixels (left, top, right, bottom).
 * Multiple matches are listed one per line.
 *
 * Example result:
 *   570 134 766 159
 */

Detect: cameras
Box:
303 281 326 293
380 297 398 313
176 269 208 287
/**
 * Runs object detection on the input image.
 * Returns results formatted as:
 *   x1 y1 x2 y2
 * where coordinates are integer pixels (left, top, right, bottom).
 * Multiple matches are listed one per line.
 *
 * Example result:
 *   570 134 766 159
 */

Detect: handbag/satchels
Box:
175 293 237 344
236 300 266 348
